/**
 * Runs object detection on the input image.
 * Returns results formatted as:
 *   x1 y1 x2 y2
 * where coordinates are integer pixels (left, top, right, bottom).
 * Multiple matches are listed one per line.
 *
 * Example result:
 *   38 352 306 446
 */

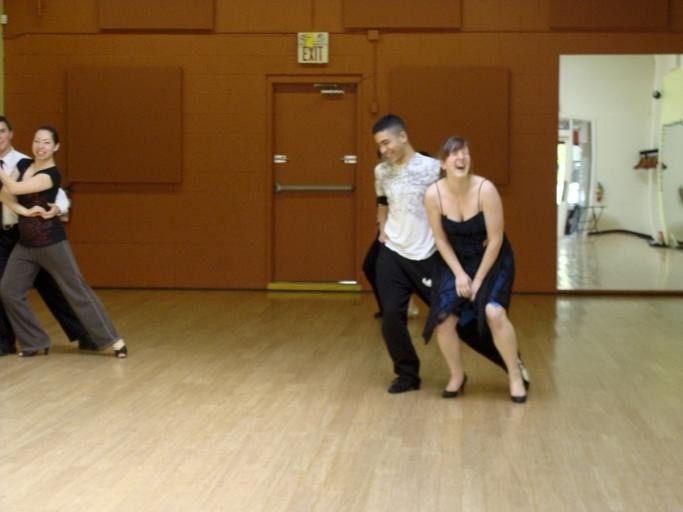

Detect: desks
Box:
568 202 606 234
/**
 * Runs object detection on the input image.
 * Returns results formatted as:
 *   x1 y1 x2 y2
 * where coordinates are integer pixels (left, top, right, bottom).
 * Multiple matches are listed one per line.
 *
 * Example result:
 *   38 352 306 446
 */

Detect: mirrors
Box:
554 51 683 295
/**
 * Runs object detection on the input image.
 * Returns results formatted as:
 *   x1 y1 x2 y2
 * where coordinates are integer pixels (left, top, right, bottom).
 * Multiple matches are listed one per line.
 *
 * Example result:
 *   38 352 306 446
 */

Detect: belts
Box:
0 223 18 230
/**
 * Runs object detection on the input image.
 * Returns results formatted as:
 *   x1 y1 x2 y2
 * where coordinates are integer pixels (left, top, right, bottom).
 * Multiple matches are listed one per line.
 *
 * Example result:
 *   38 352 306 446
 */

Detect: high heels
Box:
114 343 127 358
21 346 49 357
442 372 467 397
510 378 527 404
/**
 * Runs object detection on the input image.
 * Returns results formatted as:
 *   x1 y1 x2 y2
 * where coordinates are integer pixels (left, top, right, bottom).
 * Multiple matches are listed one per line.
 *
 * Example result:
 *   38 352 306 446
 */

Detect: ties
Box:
1 160 4 227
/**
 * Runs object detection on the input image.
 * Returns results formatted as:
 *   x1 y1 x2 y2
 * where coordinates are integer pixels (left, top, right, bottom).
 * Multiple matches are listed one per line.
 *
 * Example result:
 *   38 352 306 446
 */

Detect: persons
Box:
0 124 128 360
423 135 529 403
356 111 534 397
358 140 436 320
0 115 100 357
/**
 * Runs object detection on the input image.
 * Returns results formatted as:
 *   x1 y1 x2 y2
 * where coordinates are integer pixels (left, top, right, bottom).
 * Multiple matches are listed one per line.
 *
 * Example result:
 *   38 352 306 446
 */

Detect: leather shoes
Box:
517 356 530 387
408 306 419 318
388 378 419 393
78 339 97 351
0 345 17 357
